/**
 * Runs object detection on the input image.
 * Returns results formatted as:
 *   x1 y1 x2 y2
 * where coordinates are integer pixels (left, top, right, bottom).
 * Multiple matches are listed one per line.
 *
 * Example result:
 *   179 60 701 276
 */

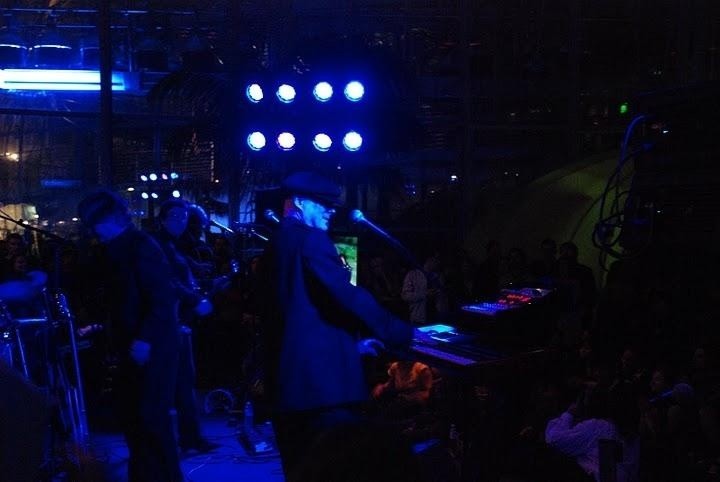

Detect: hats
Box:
281 171 347 209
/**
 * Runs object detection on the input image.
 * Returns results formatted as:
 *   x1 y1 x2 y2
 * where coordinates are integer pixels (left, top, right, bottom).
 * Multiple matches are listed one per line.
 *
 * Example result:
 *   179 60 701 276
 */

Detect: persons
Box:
76 192 219 482
0 225 32 280
367 236 720 482
257 172 440 482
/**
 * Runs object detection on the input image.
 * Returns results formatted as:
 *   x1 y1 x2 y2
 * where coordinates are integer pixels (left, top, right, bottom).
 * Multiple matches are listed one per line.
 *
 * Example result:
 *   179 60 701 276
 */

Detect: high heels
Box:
178 438 223 456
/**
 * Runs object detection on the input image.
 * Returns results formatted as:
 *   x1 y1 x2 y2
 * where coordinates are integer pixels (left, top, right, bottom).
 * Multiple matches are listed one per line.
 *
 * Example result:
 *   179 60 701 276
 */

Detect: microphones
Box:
210 219 234 234
264 208 280 224
347 209 400 245
247 228 268 242
